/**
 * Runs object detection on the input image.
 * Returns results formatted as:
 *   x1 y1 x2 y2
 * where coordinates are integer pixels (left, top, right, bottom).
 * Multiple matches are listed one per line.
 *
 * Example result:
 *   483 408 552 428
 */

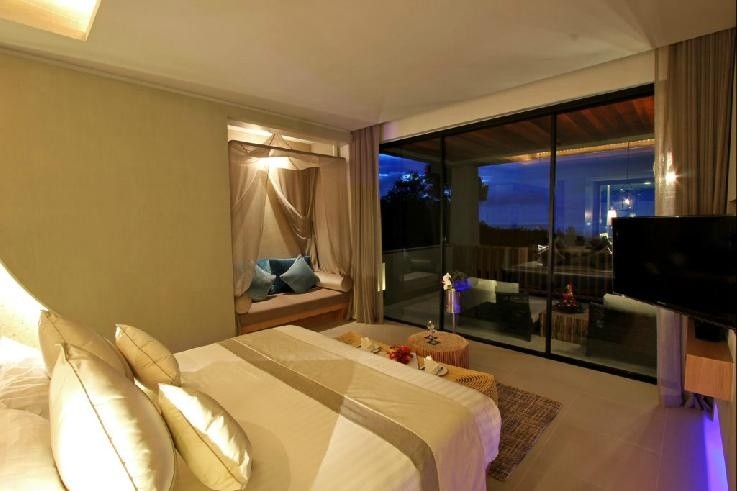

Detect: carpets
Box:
335 333 560 482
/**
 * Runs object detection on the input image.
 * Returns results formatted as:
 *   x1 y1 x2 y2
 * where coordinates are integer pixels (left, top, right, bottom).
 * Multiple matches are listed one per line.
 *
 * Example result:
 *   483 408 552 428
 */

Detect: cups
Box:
424 357 433 374
360 336 369 350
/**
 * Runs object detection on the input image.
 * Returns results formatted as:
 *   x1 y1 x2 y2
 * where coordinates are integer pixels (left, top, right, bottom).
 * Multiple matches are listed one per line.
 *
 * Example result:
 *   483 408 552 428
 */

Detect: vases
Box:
445 289 460 315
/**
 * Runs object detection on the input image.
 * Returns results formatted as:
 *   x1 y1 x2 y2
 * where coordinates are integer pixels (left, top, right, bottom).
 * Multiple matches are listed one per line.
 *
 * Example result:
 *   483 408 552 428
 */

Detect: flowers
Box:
441 271 469 292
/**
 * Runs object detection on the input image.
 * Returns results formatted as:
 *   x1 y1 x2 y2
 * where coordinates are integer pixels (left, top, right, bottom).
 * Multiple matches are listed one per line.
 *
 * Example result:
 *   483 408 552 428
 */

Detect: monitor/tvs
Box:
612 216 737 329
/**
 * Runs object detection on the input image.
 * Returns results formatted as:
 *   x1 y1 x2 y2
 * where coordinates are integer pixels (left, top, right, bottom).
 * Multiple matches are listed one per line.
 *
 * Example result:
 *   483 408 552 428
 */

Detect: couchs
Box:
461 279 534 339
586 293 657 370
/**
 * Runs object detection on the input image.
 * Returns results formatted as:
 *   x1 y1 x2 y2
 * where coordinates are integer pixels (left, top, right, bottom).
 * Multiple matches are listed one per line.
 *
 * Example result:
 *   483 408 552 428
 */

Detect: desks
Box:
539 308 586 343
408 331 469 368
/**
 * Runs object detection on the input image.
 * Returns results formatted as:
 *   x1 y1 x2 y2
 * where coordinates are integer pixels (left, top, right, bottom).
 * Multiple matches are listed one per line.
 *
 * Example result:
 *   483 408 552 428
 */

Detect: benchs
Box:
338 330 498 405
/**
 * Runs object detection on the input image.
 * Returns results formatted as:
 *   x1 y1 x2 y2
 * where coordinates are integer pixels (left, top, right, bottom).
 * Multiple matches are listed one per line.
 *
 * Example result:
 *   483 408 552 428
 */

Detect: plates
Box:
386 352 419 370
420 363 447 375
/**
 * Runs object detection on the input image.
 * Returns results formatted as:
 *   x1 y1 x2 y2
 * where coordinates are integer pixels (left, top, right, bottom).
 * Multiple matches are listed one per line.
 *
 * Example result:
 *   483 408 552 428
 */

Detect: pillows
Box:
247 255 320 301
313 272 352 292
233 296 252 315
38 312 253 491
466 276 519 295
605 295 657 316
395 249 433 272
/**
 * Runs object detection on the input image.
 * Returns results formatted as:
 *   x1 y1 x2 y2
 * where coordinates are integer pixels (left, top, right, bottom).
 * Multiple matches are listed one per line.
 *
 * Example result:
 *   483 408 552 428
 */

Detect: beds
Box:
0 266 500 491
233 285 353 335
402 271 435 292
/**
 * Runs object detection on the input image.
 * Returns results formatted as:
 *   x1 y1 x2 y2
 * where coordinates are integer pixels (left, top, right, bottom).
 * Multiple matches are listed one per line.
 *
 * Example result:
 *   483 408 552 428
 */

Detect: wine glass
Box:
430 328 437 343
427 321 436 338
